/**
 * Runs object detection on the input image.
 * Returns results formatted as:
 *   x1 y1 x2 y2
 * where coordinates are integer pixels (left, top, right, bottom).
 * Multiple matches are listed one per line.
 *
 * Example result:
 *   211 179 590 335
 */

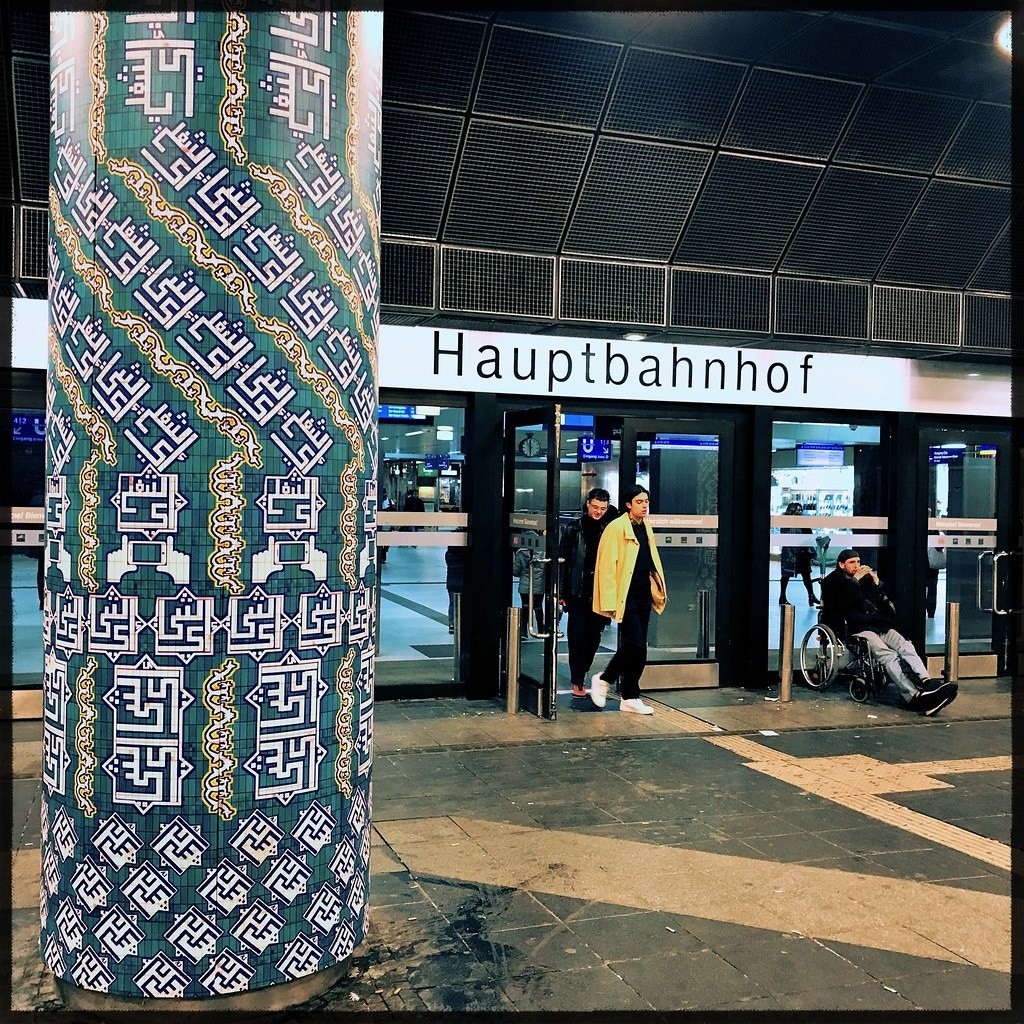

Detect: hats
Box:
837 549 859 562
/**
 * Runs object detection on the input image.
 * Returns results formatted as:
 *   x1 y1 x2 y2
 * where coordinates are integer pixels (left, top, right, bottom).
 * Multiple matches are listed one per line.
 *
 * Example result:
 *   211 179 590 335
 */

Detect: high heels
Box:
808 594 821 607
779 599 791 605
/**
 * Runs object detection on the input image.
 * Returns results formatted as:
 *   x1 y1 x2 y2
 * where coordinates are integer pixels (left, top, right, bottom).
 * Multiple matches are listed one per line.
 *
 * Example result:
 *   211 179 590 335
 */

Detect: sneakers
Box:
620 697 654 715
571 684 586 699
591 671 610 707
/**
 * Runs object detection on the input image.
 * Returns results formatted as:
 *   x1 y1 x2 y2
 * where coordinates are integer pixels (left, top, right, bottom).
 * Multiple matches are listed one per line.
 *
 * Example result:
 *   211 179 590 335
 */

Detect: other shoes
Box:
449 624 454 633
915 681 958 710
923 679 957 716
557 631 564 637
520 634 528 640
927 613 934 618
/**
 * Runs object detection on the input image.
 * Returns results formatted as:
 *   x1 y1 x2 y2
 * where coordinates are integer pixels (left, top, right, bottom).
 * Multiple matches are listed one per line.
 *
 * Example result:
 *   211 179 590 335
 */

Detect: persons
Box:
32 494 44 610
779 503 821 607
391 489 414 548
590 484 667 715
403 489 425 548
381 488 397 564
512 531 545 640
820 548 958 716
557 602 564 638
927 508 944 618
445 526 467 634
557 488 620 699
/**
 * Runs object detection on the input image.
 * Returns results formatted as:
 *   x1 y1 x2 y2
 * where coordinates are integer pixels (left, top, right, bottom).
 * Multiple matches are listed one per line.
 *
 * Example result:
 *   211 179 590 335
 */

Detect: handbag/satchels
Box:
928 546 947 570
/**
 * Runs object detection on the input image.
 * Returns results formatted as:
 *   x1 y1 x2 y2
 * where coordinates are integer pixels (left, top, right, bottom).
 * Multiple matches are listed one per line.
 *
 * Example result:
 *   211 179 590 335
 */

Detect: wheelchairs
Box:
800 577 922 707
